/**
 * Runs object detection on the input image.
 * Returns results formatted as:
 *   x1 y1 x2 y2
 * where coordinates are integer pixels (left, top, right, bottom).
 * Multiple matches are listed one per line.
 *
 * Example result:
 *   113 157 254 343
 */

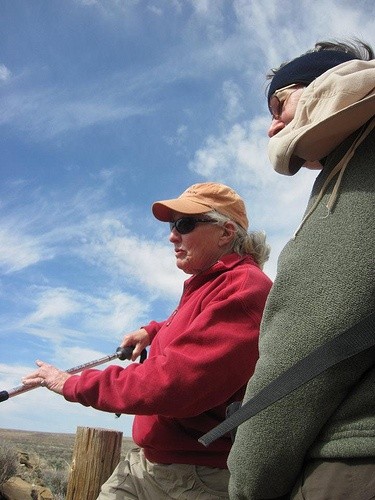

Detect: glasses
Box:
168 216 220 234
268 83 300 120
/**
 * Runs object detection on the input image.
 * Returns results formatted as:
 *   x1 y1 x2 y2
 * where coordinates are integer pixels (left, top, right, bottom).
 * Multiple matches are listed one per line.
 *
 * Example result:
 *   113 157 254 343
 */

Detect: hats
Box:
269 49 358 85
152 182 249 233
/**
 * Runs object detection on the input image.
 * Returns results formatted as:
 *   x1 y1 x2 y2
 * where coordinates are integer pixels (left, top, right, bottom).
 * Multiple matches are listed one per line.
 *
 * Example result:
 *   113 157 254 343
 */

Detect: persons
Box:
231 38 374 500
22 183 275 500
